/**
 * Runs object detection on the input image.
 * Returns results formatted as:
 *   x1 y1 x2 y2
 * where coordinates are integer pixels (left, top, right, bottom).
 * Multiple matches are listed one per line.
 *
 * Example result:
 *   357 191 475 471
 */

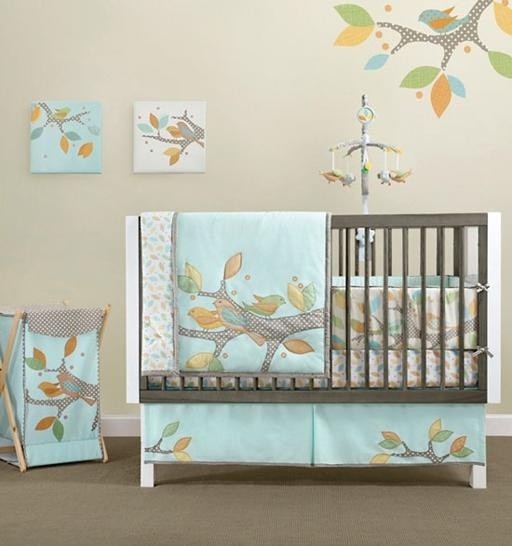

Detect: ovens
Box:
124 207 504 490
